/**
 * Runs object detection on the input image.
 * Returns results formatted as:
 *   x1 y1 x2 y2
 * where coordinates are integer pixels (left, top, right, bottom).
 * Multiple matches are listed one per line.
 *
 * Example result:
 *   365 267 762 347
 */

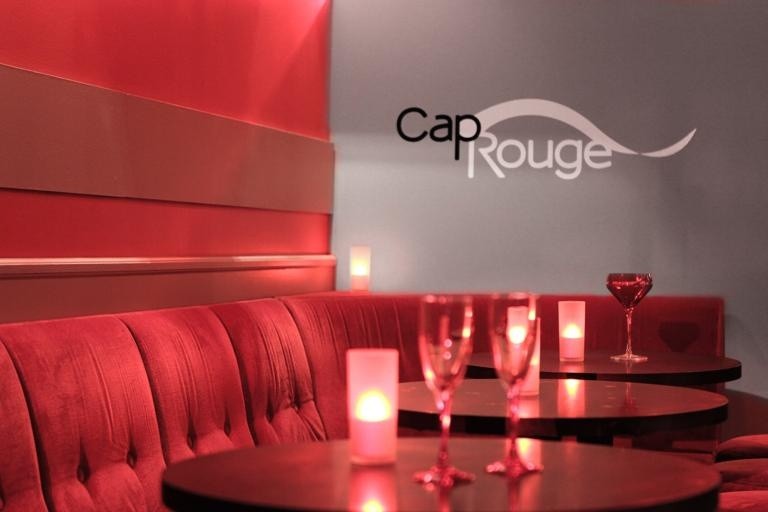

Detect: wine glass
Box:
415 288 543 487
605 272 654 365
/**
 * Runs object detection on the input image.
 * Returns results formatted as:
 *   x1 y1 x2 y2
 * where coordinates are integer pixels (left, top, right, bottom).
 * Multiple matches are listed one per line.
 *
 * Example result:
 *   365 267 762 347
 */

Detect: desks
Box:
397 377 729 445
159 438 722 511
461 350 743 393
159 349 743 511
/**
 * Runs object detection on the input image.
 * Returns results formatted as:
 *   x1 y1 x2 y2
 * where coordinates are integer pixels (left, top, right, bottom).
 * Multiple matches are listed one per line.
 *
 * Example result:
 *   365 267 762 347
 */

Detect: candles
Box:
560 336 585 359
512 332 536 392
354 395 392 454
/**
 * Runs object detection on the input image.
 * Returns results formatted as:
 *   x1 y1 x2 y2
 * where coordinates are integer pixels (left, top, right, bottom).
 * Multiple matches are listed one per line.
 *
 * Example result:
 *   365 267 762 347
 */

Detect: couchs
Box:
3 292 723 511
